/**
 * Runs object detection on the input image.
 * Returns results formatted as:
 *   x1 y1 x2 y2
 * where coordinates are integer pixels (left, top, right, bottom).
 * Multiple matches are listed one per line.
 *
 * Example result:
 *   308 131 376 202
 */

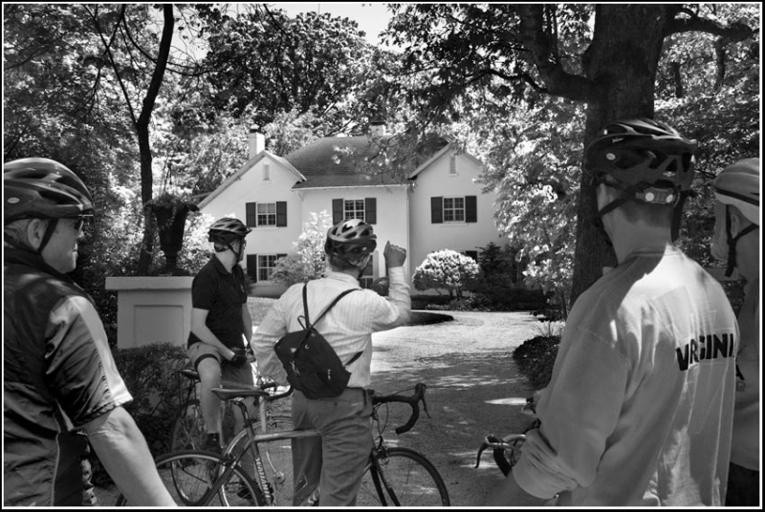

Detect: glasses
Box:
67 218 90 235
370 241 376 252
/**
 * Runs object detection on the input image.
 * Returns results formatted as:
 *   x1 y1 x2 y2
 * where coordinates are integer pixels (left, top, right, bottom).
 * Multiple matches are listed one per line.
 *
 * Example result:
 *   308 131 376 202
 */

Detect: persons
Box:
247 219 415 506
706 154 760 504
486 115 743 505
3 155 181 507
187 216 276 502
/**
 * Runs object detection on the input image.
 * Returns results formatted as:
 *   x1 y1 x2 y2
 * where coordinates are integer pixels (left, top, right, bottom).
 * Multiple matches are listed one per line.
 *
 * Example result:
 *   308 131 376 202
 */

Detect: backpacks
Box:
275 282 362 400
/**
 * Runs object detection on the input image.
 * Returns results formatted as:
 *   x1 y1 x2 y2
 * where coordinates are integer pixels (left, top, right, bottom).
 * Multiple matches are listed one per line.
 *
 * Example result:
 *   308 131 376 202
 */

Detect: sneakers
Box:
195 430 221 453
237 482 274 502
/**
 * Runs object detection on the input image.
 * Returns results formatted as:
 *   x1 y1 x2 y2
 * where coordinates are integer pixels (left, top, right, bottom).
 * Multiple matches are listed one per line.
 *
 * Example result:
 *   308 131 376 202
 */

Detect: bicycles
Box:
474 397 539 478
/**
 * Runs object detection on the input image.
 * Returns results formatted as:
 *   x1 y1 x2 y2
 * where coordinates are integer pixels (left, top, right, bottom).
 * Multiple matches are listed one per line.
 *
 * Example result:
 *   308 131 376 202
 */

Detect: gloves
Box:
229 347 247 367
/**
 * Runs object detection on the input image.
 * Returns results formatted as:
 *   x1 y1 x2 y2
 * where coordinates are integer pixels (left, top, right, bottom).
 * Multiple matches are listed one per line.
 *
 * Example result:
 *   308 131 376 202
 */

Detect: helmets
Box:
325 218 377 261
4 157 94 217
583 115 699 205
713 157 758 223
208 218 251 243
